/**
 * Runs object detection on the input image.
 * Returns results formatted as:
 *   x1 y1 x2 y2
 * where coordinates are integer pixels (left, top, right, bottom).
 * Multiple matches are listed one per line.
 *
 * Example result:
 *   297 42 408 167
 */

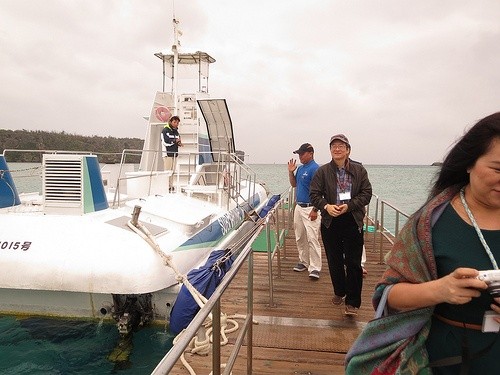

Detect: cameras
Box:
464 270 500 295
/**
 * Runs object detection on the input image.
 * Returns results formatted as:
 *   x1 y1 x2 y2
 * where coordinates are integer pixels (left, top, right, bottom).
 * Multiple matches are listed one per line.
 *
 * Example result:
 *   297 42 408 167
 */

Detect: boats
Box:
0 0 283 362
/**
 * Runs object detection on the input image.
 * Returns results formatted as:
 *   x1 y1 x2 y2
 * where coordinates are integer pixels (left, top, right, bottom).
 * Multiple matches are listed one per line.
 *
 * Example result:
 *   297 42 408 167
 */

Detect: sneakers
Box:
293 263 307 272
331 295 346 307
344 305 358 315
309 269 320 279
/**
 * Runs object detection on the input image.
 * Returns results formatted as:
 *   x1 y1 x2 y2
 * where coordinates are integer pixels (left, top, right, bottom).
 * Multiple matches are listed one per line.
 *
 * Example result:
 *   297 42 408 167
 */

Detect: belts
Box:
298 203 313 208
431 314 484 330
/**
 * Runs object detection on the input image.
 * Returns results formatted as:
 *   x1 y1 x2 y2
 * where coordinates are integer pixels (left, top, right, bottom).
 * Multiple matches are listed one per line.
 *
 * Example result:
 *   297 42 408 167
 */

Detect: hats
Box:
292 143 314 155
329 134 349 144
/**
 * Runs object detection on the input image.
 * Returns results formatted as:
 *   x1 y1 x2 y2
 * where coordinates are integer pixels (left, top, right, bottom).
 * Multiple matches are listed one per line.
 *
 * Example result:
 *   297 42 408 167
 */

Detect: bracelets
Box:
312 208 318 213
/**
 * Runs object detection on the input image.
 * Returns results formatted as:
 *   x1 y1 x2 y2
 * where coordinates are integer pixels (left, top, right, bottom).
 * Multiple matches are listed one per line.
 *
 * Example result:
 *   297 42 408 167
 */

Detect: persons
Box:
310 134 373 316
372 112 500 375
288 143 322 279
161 116 184 190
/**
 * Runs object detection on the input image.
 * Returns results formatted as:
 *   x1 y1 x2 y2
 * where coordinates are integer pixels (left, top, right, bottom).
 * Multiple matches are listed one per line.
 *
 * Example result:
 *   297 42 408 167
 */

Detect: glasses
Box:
332 144 345 149
